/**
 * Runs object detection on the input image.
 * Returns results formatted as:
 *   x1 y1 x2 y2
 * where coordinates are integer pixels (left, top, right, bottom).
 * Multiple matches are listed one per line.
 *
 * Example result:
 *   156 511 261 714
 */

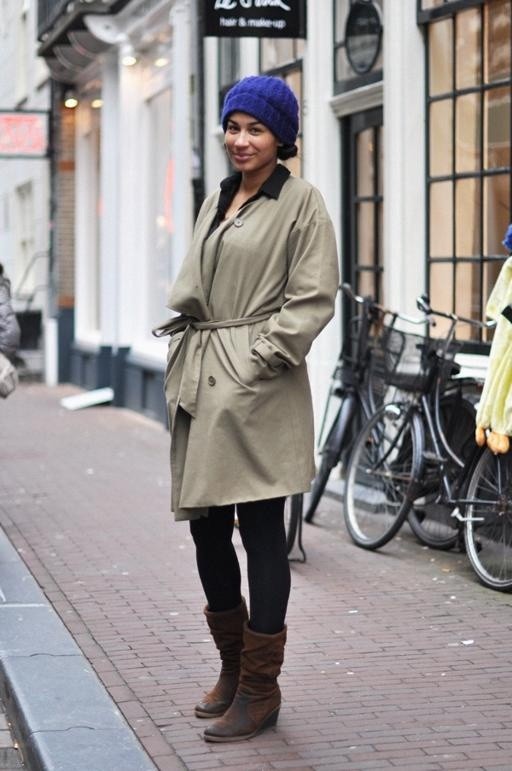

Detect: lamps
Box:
153 44 169 67
86 88 104 109
63 88 78 108
122 43 136 66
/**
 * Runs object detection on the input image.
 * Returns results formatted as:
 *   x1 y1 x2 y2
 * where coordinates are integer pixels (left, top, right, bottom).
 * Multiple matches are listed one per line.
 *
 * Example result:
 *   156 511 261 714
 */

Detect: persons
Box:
159 76 340 742
0 263 21 400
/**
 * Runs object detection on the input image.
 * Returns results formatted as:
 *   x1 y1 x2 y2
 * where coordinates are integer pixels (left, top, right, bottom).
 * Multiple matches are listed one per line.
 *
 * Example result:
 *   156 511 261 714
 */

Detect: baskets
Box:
380 322 464 395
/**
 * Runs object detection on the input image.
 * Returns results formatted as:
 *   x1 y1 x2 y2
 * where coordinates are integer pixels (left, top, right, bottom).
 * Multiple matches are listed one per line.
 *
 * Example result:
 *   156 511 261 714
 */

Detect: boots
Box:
203 619 287 744
192 596 250 718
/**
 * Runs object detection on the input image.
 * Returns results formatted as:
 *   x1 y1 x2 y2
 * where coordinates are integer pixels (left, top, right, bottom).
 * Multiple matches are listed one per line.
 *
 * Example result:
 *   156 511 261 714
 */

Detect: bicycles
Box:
304 285 491 548
347 292 512 597
228 487 308 566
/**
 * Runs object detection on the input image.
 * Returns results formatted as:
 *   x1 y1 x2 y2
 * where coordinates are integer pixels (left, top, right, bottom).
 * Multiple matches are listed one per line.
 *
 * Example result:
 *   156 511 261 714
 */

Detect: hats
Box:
221 75 299 146
